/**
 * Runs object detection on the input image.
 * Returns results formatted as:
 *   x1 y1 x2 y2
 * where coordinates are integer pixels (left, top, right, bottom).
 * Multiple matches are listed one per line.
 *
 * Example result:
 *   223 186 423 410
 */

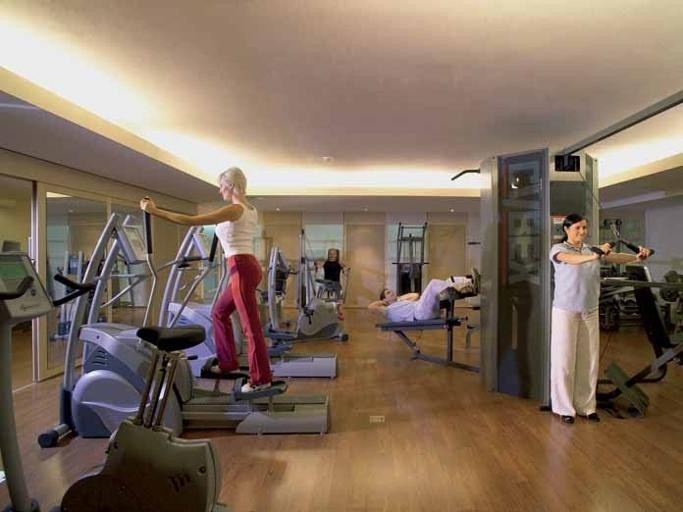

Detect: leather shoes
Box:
562 415 575 424
577 412 600 422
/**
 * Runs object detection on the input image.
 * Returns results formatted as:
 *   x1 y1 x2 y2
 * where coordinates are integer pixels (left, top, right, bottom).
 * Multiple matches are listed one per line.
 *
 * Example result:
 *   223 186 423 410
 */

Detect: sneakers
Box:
241 380 272 392
210 365 240 373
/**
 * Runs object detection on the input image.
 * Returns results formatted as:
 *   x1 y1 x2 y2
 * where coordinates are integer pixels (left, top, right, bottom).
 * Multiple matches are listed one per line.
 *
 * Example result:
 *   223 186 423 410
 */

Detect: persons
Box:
307 247 351 319
367 268 480 323
664 271 683 298
139 166 273 394
548 214 651 424
